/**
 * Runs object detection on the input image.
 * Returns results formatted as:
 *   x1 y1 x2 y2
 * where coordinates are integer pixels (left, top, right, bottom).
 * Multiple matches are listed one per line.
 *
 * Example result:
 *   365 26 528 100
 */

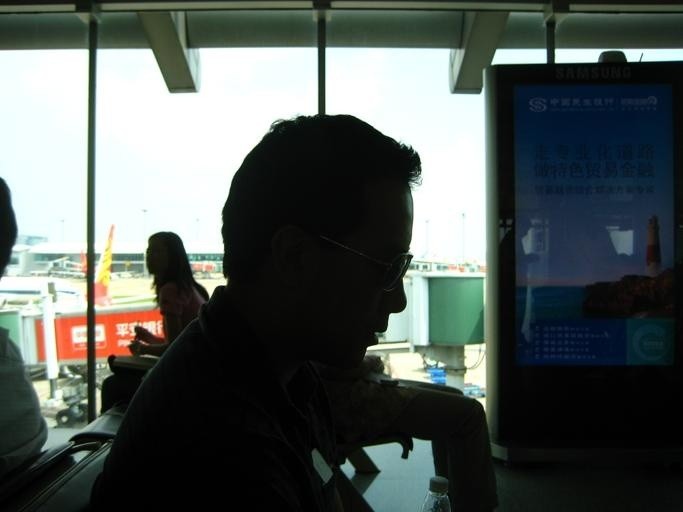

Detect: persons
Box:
102 114 498 512
102 231 209 416
323 356 498 512
0 179 48 479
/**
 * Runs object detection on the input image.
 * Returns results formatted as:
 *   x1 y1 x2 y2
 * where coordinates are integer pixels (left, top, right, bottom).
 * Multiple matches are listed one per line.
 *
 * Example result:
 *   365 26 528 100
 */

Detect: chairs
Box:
111 283 229 405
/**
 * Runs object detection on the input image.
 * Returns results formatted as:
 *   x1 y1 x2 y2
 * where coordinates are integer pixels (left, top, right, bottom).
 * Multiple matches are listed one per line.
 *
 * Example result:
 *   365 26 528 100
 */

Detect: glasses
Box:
317 234 413 295
